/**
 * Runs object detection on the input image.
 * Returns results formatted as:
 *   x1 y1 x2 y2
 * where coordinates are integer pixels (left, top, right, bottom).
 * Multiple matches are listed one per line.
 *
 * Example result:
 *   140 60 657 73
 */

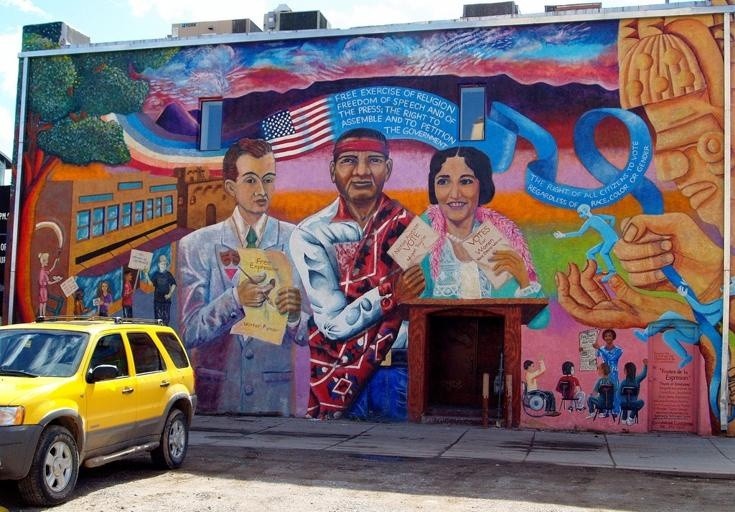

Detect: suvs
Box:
0 312 200 508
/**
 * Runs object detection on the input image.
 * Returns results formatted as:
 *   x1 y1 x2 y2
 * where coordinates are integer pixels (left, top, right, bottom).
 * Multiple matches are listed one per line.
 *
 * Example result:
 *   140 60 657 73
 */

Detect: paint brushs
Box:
238 266 274 305
